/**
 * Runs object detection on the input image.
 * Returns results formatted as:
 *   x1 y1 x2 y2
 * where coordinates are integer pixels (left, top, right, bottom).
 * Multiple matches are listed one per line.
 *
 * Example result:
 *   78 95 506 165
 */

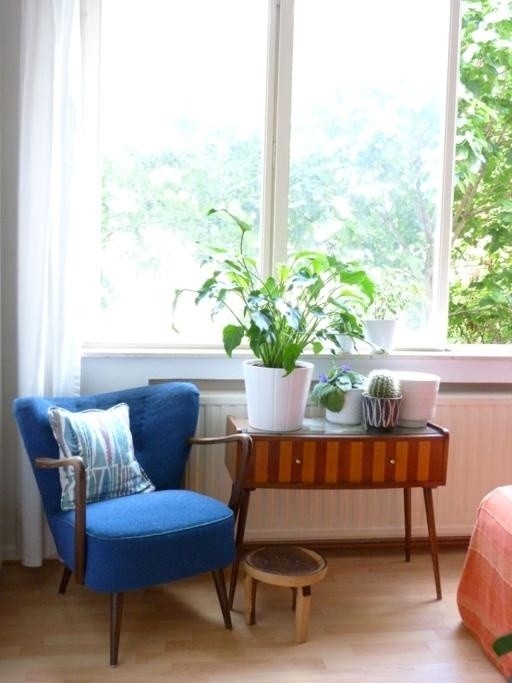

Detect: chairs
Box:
14 382 252 668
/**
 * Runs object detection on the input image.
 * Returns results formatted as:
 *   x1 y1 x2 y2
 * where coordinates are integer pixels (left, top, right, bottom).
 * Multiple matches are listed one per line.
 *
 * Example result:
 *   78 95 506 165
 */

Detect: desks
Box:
225 415 448 611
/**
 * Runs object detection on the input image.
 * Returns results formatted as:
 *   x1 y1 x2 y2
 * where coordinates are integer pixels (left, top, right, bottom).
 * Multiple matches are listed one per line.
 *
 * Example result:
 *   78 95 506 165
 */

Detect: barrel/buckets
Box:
242 360 313 434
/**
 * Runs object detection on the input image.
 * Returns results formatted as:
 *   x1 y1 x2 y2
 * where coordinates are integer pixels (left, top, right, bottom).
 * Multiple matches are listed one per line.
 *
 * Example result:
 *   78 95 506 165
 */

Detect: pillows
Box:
45 402 157 512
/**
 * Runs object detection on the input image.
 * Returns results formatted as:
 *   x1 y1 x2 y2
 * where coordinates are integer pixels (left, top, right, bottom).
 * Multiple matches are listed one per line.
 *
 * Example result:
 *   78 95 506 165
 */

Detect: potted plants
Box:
362 368 402 428
365 279 402 351
328 304 363 352
310 366 367 427
172 209 386 434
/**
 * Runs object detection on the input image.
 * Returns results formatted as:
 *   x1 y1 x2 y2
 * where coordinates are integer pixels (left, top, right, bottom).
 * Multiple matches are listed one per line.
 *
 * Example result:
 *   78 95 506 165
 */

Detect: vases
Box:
399 372 441 427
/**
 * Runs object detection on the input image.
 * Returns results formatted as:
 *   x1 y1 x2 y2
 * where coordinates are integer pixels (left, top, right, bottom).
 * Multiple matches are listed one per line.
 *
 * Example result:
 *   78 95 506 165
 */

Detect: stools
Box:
242 545 327 642
